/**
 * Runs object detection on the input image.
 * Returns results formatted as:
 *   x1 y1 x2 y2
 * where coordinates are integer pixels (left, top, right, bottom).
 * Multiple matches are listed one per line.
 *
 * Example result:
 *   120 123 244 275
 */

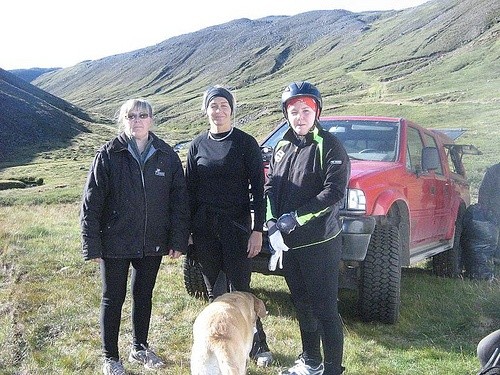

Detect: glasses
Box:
125 113 150 120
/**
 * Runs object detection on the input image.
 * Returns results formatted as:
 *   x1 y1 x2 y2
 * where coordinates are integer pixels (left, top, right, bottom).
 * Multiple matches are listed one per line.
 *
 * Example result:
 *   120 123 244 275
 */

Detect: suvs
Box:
181 116 484 324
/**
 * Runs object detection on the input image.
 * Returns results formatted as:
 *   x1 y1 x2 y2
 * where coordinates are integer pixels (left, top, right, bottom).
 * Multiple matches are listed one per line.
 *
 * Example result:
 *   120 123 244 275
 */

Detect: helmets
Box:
281 82 322 114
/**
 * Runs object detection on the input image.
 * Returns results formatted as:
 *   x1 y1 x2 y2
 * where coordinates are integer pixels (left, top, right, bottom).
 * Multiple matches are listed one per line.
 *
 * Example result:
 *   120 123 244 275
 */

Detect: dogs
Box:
191 291 265 375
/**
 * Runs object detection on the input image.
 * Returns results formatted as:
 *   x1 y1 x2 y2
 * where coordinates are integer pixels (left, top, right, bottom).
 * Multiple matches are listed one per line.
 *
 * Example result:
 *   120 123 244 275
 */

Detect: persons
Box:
80 98 190 375
184 86 273 365
264 82 351 375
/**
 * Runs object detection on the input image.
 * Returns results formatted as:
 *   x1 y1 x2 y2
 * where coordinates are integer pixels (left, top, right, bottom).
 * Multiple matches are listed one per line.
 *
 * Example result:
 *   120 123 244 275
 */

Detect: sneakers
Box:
129 349 164 369
279 358 324 375
103 358 125 375
257 352 272 366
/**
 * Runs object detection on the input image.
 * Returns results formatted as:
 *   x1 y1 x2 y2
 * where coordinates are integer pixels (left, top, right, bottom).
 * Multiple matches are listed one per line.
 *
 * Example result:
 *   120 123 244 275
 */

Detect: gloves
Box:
276 212 296 235
268 224 290 271
